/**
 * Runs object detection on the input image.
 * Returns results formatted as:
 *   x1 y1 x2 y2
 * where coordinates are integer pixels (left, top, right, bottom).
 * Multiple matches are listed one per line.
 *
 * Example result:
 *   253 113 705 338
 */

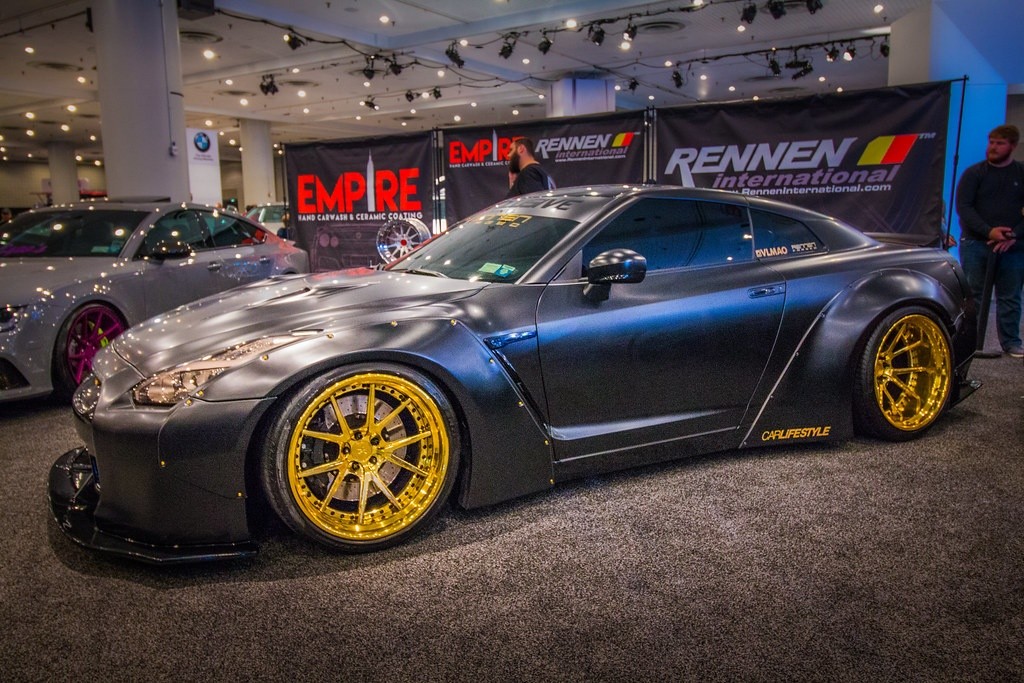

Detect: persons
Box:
941 200 958 248
276 212 291 240
507 138 556 199
954 124 1024 358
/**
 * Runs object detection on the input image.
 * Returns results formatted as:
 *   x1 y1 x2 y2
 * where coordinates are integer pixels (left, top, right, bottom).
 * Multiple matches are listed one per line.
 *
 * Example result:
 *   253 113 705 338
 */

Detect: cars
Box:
241 204 290 238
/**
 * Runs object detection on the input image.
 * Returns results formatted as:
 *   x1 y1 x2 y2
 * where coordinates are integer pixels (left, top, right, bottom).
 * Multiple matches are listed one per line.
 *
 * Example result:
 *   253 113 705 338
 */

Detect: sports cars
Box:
49 184 984 565
0 202 308 403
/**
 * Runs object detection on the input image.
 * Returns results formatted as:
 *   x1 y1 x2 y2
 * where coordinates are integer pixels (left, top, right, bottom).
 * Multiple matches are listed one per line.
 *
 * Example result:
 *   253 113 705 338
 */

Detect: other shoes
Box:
1005 346 1024 358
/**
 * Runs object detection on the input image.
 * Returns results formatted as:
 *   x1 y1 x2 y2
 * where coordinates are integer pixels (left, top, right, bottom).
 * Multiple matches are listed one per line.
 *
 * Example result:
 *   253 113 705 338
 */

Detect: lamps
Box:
624 22 638 42
768 59 781 77
499 44 513 59
806 0 823 15
844 41 856 62
827 43 840 64
590 27 605 46
365 101 381 111
287 33 301 51
259 81 279 97
672 70 682 89
740 0 758 25
767 0 787 21
405 94 415 102
433 89 442 98
784 48 813 82
538 37 552 55
879 35 890 57
445 49 464 68
